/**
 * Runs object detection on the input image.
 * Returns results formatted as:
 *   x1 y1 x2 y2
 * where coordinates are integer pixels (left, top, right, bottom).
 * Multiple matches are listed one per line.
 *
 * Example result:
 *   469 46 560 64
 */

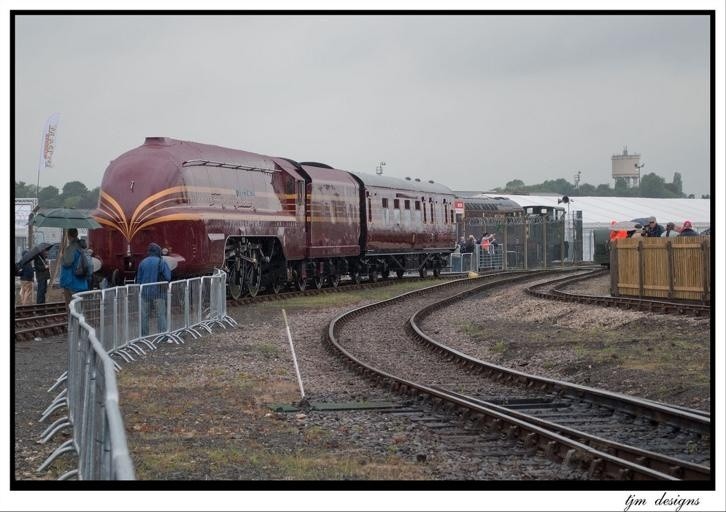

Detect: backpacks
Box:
72 248 94 277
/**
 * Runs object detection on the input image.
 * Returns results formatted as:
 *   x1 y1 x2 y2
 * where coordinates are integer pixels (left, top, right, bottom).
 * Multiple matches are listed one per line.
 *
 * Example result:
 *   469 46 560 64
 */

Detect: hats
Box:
684 221 692 229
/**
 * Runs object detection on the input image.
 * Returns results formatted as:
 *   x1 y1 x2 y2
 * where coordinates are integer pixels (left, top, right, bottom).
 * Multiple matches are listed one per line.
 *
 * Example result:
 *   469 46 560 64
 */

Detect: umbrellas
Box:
24 205 105 230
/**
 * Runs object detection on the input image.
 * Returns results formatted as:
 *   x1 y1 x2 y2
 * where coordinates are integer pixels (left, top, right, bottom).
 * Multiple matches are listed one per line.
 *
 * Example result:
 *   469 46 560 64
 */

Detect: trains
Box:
91 134 525 300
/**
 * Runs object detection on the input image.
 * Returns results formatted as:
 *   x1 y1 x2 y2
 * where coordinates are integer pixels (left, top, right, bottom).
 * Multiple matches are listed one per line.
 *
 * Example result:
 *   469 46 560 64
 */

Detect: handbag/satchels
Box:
159 279 168 293
38 272 50 280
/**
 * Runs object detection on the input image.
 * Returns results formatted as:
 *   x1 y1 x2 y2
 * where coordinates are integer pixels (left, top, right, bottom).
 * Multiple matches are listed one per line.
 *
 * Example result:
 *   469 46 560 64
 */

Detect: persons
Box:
17 228 194 337
608 216 710 240
450 232 499 272
